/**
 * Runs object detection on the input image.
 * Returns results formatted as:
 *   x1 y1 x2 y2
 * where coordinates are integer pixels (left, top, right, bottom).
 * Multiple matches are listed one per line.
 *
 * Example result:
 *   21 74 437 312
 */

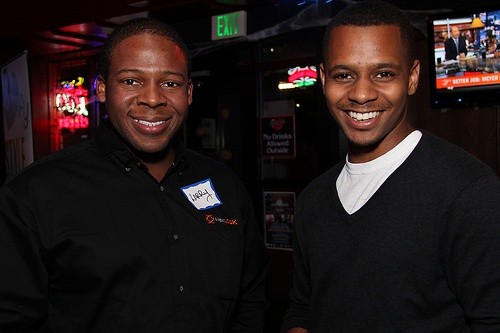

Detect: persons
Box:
444 27 468 60
0 18 265 333
282 0 500 333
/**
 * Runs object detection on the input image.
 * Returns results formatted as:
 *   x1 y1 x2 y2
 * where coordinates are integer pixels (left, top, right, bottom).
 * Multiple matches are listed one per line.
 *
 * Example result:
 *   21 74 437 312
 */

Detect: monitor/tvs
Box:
426 6 500 111
